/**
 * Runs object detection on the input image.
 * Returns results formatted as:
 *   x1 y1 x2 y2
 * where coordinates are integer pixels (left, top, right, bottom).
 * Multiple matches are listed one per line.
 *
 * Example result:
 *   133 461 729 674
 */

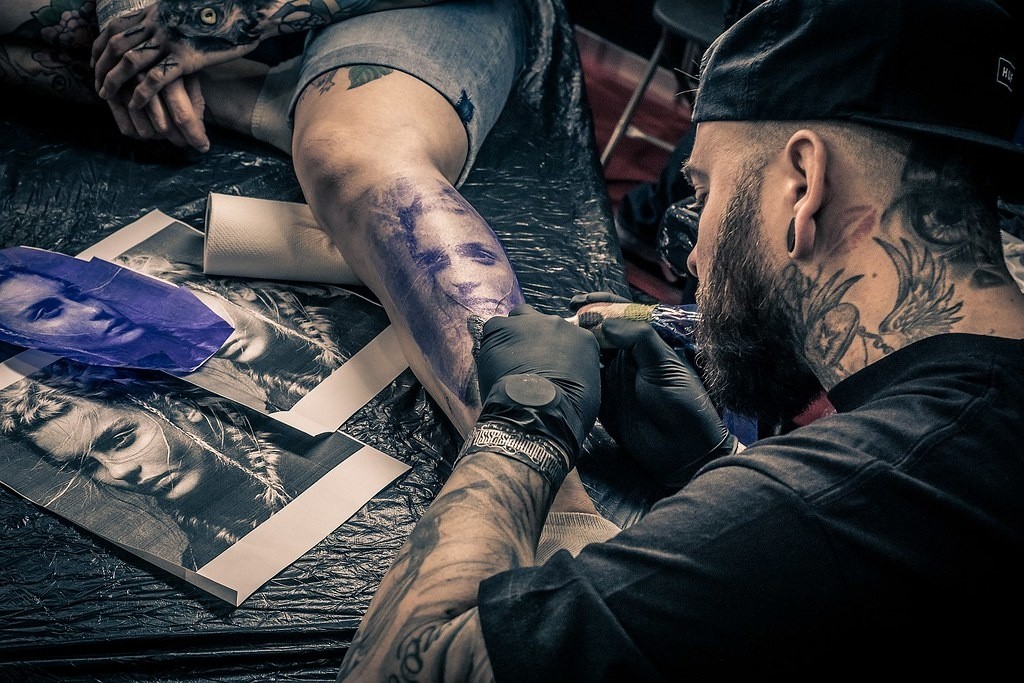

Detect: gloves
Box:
571 291 741 489
471 302 602 474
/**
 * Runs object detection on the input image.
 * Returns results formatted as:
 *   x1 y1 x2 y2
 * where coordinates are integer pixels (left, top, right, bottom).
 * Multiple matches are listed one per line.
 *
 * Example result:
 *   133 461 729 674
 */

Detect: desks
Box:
0 2 662 680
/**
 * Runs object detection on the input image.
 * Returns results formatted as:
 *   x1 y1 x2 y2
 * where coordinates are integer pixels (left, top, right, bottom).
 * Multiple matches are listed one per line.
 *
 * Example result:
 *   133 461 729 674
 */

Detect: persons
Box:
0 0 629 568
336 2 1024 682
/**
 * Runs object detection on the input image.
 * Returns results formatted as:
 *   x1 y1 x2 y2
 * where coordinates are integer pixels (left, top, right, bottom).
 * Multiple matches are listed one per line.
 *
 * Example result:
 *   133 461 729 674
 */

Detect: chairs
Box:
597 1 726 184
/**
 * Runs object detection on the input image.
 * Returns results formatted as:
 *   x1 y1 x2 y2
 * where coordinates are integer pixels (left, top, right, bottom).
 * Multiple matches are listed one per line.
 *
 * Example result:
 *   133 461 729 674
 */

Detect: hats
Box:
693 1 1023 207
